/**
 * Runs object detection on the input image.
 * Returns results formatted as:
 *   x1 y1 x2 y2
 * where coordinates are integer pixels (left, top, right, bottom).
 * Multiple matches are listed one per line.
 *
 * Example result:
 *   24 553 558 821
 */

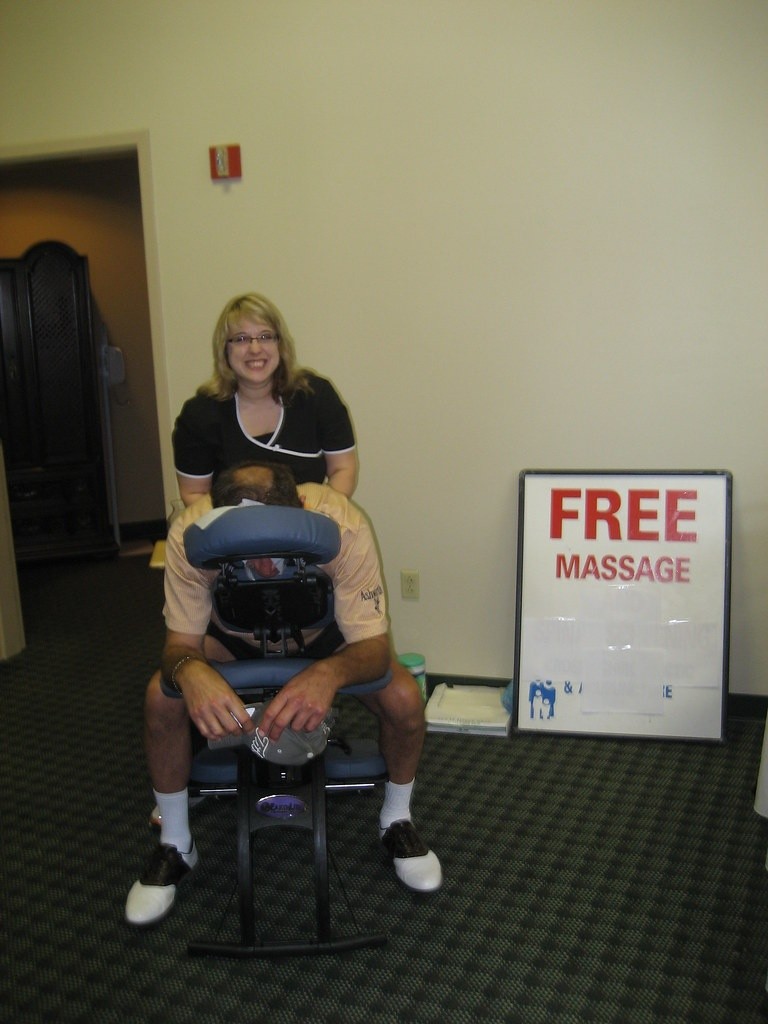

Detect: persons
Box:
124 460 444 928
149 291 359 830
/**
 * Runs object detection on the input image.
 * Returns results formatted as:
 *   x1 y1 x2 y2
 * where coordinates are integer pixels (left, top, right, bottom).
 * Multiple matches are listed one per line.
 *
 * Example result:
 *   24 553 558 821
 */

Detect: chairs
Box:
159 505 395 959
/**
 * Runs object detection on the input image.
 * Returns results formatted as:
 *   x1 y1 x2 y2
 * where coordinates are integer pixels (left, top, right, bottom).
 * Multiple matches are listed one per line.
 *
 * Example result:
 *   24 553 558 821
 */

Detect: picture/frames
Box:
511 468 735 744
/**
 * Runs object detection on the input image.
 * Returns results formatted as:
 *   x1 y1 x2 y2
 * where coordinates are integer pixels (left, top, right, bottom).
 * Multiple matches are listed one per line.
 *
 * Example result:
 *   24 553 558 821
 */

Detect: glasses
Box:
226 333 281 347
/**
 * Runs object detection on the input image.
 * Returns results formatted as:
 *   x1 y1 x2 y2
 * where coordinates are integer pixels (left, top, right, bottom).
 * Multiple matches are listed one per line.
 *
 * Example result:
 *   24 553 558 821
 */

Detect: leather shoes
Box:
125 834 200 923
379 817 444 894
148 794 207 827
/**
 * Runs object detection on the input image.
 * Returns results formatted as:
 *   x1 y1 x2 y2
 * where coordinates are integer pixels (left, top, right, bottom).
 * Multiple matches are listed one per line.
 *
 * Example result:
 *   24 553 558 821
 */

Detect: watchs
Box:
170 654 200 696
